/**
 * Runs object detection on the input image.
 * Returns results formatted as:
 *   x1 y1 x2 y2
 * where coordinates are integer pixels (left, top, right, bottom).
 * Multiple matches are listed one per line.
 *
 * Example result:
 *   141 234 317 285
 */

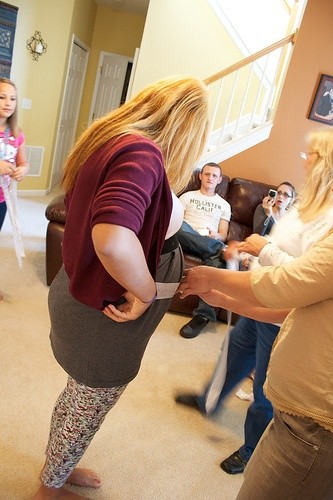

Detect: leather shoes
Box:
175 393 199 410
220 450 246 474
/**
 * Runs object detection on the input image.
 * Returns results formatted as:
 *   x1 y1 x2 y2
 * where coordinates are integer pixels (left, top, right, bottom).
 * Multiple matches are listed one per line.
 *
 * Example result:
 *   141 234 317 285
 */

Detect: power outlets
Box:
22 98 33 110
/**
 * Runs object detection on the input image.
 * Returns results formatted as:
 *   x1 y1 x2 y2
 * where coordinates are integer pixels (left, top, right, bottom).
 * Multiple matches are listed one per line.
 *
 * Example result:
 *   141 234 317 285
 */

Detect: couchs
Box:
45 169 303 326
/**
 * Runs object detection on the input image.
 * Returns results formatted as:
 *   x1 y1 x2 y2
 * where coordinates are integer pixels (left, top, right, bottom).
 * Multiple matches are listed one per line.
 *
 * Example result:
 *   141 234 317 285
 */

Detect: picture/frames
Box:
308 74 333 125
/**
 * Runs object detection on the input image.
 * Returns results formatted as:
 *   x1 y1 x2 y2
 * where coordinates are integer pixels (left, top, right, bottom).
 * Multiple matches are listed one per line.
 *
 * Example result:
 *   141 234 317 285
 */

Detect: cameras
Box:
268 189 277 203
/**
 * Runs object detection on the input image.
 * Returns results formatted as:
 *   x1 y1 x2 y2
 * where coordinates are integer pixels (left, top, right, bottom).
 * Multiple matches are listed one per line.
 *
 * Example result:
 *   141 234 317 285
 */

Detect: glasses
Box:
277 191 292 198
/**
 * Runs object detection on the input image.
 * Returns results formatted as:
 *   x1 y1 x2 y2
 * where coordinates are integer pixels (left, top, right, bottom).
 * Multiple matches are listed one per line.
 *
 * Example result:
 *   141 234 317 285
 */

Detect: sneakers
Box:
179 313 210 338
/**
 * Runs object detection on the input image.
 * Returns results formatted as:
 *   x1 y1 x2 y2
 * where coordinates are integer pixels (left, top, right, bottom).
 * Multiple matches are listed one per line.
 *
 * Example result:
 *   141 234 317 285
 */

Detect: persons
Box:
33 76 209 500
0 77 29 300
176 128 333 500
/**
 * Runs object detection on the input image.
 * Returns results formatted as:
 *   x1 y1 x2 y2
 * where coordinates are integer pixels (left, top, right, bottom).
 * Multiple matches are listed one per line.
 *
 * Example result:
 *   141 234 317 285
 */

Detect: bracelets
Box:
142 290 158 304
